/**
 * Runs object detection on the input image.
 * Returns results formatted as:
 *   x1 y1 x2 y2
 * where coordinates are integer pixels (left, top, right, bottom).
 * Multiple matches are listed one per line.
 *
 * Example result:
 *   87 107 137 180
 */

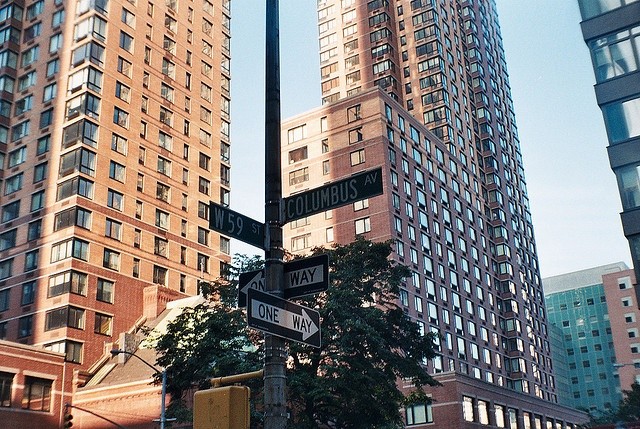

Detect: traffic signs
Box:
239 253 328 306
209 200 266 250
281 165 383 228
248 287 322 345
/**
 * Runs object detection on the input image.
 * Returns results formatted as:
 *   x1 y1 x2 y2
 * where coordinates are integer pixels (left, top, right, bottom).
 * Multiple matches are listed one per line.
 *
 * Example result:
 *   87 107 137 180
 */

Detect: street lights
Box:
110 350 167 429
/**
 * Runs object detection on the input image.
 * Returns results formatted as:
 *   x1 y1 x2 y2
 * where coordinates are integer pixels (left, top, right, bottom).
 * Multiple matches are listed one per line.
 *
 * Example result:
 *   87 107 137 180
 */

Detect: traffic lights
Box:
63 410 73 428
192 386 251 429
